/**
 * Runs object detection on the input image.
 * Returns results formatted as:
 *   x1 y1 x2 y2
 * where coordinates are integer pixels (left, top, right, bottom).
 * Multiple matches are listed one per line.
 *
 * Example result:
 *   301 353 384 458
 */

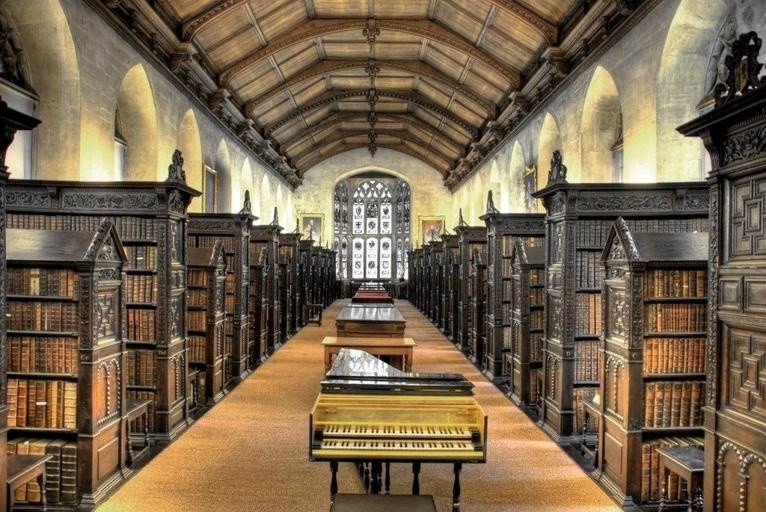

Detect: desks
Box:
320 282 417 373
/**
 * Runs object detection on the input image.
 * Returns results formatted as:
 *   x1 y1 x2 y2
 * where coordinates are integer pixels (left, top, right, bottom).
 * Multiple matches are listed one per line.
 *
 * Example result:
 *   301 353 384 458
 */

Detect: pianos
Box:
312 347 489 512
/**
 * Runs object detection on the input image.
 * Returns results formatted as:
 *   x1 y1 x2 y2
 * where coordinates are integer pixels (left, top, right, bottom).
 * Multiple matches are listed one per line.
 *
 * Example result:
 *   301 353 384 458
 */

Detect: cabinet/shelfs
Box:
0 150 342 512
405 86 766 512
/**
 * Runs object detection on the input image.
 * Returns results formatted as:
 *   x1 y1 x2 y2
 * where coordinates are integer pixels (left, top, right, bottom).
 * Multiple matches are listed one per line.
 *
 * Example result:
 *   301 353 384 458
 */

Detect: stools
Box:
330 493 438 512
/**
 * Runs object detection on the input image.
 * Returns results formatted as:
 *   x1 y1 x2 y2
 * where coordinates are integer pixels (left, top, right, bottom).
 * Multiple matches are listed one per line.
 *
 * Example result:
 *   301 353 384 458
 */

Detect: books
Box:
6 213 156 242
188 236 234 251
502 258 513 350
468 243 488 298
577 220 708 247
573 250 602 434
121 246 159 434
185 269 209 363
530 270 545 403
3 267 81 428
249 269 256 340
503 235 545 257
226 256 236 355
640 268 708 501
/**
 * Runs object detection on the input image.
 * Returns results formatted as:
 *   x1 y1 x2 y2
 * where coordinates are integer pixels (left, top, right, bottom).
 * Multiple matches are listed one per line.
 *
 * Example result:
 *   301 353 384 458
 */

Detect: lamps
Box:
359 17 379 160
